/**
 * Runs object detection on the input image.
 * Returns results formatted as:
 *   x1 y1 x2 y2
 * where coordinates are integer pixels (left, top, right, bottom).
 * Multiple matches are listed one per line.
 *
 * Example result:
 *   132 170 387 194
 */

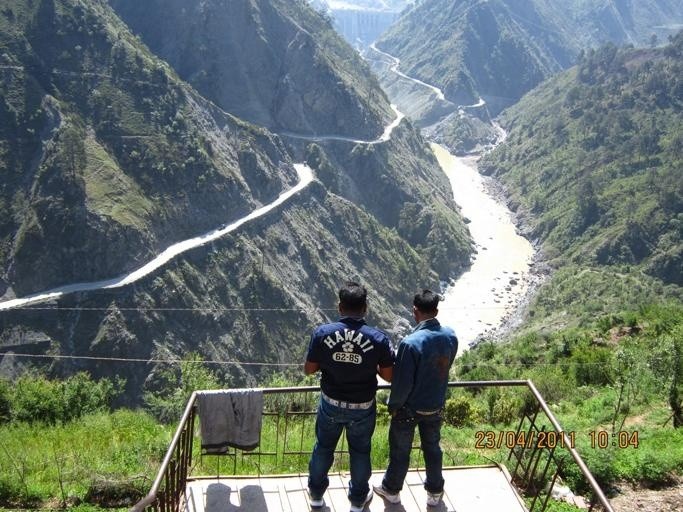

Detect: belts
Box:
320 393 374 410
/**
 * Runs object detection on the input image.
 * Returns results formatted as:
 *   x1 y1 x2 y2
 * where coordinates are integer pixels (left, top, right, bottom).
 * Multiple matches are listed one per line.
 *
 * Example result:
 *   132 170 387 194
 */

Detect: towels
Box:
194 386 264 455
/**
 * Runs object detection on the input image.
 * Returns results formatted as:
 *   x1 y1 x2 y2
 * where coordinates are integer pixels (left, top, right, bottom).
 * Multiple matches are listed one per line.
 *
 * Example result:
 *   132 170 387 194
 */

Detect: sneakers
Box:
427 490 443 506
349 483 374 512
305 484 323 507
374 480 401 504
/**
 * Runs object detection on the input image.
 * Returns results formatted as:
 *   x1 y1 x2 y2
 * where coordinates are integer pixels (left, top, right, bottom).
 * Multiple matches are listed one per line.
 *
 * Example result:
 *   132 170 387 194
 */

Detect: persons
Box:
302 282 396 511
372 289 458 507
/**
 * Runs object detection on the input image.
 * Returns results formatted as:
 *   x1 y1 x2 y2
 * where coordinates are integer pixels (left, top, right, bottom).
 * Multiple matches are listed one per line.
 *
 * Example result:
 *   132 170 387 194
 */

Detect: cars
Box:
217 222 226 232
